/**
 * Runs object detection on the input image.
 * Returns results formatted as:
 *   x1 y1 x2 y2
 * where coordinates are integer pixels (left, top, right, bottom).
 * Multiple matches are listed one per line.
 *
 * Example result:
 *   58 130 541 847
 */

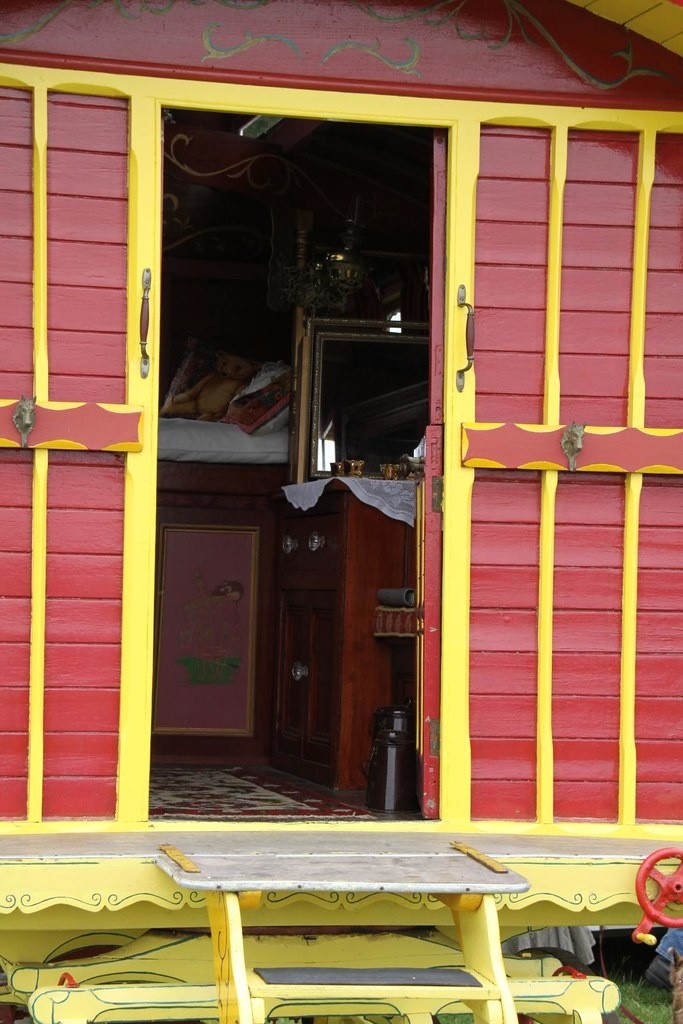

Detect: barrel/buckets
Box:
367 697 415 815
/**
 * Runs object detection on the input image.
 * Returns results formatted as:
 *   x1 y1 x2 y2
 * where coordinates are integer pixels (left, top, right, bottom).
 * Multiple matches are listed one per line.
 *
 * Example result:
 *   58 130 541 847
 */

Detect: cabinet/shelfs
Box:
158 418 290 765
270 476 419 791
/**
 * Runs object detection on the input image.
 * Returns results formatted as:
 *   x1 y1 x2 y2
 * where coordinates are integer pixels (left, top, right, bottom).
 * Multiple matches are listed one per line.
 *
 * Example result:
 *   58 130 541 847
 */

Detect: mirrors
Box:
292 315 429 485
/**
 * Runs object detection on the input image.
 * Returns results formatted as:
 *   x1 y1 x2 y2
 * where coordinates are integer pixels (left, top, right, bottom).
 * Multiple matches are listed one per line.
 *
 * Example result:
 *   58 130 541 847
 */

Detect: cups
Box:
330 459 364 477
380 462 401 480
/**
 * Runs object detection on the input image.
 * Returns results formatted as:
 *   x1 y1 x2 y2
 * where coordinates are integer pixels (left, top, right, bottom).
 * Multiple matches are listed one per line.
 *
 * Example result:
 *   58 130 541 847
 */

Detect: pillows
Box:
159 334 262 423
215 358 292 437
